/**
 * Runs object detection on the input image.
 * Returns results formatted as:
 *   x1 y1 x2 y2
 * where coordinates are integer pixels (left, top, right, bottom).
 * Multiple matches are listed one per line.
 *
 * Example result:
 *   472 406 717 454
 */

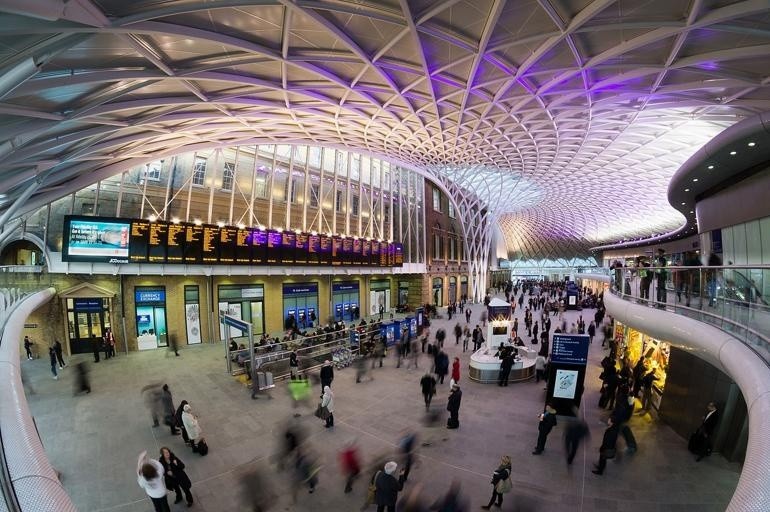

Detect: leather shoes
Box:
482 506 490 510
187 503 192 507
172 429 198 452
174 496 182 504
529 444 606 476
495 503 500 508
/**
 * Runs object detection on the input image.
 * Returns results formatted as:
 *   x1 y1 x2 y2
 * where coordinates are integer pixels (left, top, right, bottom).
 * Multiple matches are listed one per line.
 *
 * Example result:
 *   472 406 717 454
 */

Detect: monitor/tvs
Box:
493 326 507 335
61 214 130 264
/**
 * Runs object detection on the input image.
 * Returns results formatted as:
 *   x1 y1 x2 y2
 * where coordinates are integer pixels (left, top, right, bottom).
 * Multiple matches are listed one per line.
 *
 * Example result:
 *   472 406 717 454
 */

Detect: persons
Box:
135 450 170 512
53 340 68 373
147 383 203 453
701 401 721 450
72 361 92 398
164 331 180 359
158 446 195 508
608 252 726 310
90 328 116 363
23 335 34 362
48 347 59 381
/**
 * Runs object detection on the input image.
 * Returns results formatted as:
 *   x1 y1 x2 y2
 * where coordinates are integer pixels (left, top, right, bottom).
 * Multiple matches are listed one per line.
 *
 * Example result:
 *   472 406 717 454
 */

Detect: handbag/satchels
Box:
495 478 513 494
447 418 459 427
368 470 381 504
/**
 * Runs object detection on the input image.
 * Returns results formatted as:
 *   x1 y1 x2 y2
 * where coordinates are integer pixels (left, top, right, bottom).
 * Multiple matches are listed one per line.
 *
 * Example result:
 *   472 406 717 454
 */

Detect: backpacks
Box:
198 439 208 456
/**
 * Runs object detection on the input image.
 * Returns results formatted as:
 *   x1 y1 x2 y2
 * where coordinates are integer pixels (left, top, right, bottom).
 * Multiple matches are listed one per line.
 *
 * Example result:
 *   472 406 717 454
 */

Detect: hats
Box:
501 455 511 463
183 404 190 411
384 461 398 475
